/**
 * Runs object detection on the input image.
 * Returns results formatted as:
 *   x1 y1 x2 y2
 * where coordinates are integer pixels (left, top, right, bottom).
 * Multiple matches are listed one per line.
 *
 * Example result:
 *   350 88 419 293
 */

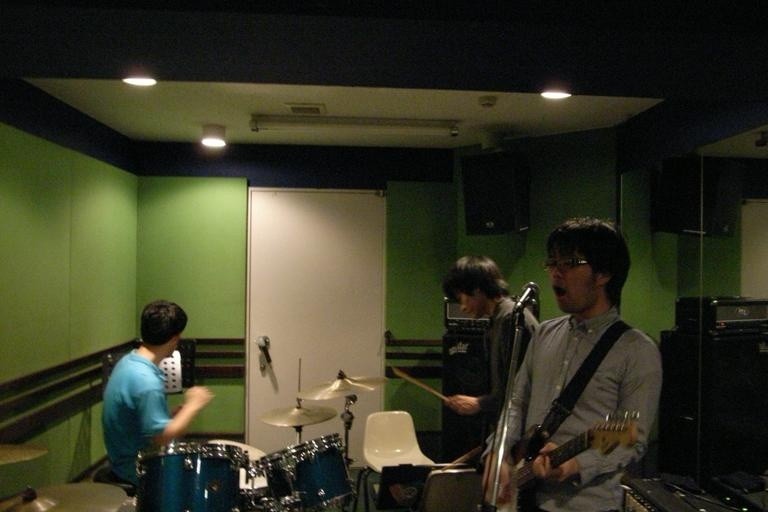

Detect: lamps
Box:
250 115 460 139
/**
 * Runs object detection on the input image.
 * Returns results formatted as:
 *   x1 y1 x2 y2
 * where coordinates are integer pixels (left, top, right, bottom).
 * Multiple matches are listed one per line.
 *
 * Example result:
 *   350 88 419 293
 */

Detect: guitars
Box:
494 410 639 511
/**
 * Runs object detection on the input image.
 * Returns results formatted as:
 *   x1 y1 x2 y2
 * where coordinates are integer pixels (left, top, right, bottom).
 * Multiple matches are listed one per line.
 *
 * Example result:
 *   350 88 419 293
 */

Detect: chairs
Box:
353 410 435 511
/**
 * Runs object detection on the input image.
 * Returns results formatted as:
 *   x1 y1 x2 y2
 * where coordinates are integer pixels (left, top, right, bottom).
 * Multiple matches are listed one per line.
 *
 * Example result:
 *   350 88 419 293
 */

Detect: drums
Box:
135 442 243 512
201 438 269 512
260 433 353 512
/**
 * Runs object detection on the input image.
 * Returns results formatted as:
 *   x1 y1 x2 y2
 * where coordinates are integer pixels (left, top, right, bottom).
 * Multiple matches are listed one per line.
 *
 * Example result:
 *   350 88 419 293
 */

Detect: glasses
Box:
544 258 588 267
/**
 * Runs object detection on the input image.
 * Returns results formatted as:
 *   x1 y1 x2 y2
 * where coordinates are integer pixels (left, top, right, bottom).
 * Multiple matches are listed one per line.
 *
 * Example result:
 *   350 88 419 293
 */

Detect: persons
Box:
444 255 540 467
102 299 216 487
480 217 665 512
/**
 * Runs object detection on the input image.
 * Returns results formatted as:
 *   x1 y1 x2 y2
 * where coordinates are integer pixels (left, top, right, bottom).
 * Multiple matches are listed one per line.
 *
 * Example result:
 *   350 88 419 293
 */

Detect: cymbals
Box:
0 442 49 465
0 482 128 512
295 376 389 401
259 406 337 428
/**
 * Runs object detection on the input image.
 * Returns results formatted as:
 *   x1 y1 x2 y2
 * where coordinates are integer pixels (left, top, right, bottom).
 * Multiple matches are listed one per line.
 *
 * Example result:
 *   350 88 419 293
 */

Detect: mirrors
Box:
621 124 766 512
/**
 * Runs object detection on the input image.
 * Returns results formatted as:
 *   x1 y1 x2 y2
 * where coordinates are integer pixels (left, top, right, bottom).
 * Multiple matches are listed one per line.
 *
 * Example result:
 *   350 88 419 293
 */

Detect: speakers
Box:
650 155 743 239
462 153 529 236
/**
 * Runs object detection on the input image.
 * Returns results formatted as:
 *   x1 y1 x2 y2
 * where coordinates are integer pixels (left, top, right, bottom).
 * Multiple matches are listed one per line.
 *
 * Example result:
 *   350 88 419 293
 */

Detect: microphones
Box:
512 281 540 315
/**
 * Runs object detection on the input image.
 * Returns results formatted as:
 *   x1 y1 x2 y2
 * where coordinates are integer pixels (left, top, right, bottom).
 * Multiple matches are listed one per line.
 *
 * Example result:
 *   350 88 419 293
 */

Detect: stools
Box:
93 467 136 497
